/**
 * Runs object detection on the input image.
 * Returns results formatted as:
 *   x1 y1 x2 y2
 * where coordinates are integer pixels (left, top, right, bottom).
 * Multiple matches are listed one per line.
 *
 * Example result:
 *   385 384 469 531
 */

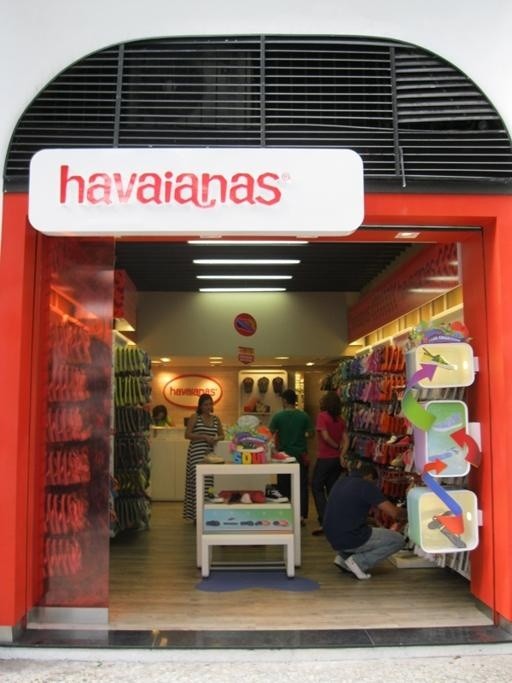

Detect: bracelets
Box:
337 442 341 451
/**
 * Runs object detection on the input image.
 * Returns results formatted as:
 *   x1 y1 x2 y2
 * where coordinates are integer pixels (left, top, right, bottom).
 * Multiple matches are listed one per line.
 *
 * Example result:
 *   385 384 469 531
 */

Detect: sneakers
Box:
208 486 224 503
418 346 457 370
334 555 352 572
240 491 253 503
271 450 296 462
265 484 289 503
204 450 224 463
312 527 325 536
228 493 241 503
344 556 372 580
432 413 463 431
251 490 266 502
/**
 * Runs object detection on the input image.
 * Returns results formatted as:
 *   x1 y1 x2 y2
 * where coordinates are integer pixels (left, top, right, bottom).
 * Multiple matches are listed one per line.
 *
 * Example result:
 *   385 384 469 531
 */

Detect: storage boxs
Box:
113 269 138 332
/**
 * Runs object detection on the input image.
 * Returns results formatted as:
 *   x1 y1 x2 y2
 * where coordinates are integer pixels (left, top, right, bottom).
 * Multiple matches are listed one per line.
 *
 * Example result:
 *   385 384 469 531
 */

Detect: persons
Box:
268 389 315 527
183 394 225 524
151 405 175 436
311 390 349 536
323 466 405 580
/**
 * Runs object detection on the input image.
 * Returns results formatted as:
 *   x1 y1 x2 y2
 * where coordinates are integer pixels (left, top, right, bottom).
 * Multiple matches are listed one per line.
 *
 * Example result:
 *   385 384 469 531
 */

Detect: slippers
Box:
40 323 151 579
324 345 415 543
440 526 467 547
428 510 454 529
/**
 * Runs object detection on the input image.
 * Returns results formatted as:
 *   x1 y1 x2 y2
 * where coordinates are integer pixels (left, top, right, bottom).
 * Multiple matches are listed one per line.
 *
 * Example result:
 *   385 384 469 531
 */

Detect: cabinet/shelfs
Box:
238 369 288 427
30 290 110 609
195 458 302 578
334 284 464 568
113 329 153 536
